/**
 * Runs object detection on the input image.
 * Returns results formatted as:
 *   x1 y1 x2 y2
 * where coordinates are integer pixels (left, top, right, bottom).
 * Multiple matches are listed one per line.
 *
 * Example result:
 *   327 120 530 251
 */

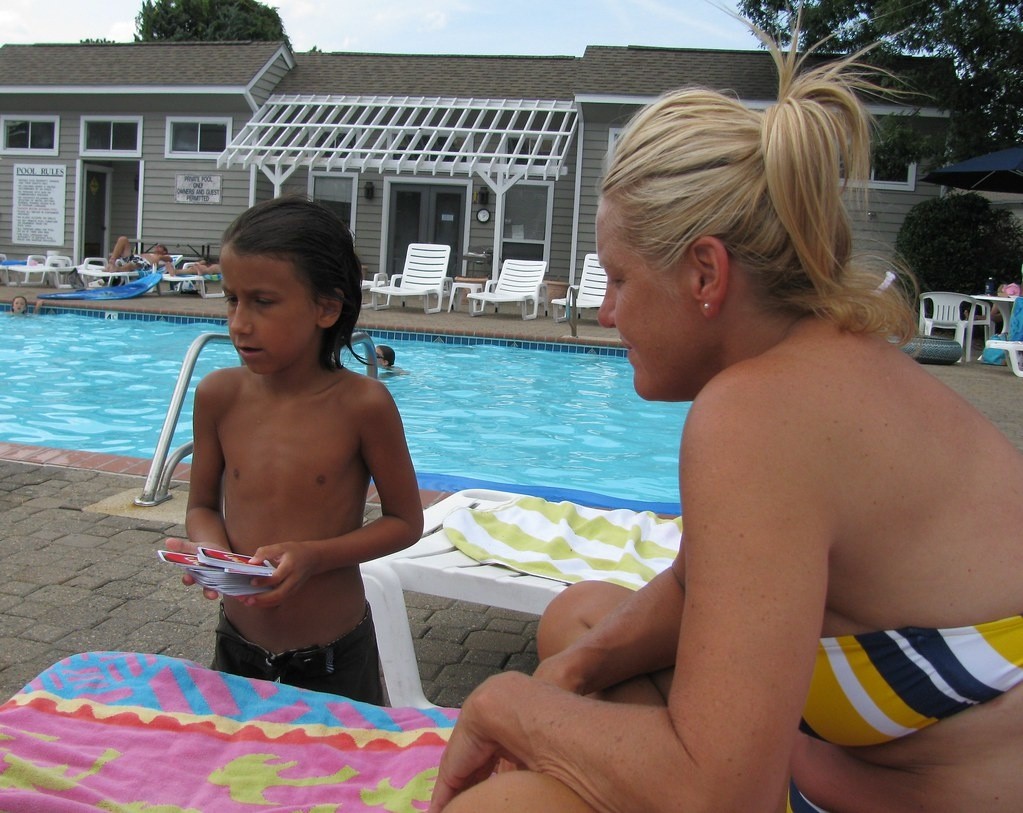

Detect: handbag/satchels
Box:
980 333 1008 366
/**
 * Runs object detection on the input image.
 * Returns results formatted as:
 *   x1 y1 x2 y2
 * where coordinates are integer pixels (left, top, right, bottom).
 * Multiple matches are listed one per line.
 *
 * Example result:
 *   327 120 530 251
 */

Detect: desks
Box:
971 294 1017 360
447 282 483 316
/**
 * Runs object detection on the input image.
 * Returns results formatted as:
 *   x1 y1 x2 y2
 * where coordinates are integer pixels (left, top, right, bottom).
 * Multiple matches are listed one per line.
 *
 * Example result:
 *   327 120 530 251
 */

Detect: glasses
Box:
377 353 383 359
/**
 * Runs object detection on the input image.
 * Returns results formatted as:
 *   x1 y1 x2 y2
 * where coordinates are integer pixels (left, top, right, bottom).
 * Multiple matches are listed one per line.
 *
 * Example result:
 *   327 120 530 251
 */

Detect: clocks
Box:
477 208 491 224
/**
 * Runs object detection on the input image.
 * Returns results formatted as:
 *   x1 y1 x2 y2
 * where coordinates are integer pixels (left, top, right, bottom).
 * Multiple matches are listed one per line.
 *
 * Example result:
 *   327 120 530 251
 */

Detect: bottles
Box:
985 278 994 296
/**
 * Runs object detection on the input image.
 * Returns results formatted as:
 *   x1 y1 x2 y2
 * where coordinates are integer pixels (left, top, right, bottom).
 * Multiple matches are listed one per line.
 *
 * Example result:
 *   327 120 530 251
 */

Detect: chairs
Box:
919 291 991 363
0 650 463 813
359 487 684 711
0 254 227 299
362 272 390 310
551 253 608 326
369 242 454 315
466 258 549 321
984 296 1023 378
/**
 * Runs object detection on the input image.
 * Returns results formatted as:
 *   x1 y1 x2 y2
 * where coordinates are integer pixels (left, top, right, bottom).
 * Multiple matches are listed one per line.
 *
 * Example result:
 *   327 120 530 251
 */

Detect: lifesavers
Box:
885 334 963 364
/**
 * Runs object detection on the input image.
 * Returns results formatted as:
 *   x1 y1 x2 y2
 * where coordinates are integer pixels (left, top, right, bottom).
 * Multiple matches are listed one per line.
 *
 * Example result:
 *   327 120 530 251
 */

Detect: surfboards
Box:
37 273 161 300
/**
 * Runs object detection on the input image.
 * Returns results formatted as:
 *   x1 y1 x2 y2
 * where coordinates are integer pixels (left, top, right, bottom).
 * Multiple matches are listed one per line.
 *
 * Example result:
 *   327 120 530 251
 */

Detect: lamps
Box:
364 181 375 201
477 187 490 205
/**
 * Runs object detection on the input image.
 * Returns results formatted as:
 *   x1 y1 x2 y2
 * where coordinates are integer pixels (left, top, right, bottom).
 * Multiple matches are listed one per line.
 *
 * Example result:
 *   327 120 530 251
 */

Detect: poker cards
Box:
158 547 276 596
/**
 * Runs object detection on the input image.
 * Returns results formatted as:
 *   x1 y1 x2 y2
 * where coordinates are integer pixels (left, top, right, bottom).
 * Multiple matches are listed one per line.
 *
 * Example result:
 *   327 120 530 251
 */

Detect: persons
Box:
423 2 1022 812
5 235 223 317
374 345 396 368
164 195 426 707
